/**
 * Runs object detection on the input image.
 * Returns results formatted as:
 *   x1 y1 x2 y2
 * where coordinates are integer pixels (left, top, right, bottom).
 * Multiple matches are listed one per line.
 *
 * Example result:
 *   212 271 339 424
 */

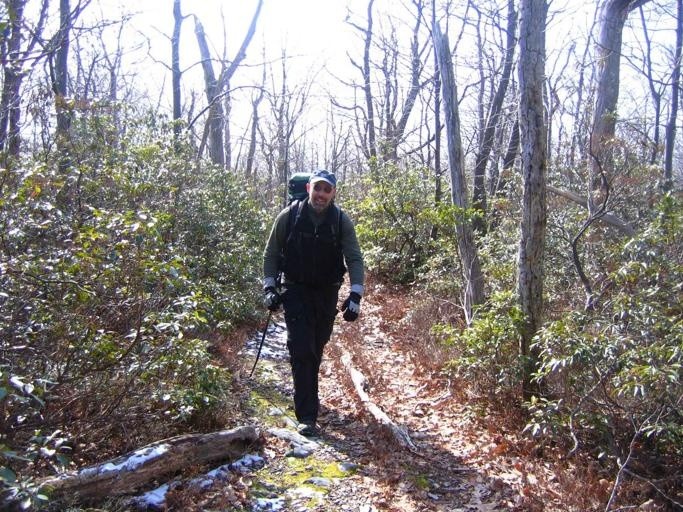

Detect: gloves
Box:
263 275 281 312
341 284 363 322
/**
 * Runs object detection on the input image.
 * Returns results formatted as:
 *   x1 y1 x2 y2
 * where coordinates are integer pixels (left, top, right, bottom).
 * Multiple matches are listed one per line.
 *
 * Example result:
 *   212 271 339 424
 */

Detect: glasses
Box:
314 185 332 193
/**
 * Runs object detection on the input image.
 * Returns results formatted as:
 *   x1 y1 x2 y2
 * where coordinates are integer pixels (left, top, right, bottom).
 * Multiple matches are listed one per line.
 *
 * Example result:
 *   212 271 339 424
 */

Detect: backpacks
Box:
285 174 342 255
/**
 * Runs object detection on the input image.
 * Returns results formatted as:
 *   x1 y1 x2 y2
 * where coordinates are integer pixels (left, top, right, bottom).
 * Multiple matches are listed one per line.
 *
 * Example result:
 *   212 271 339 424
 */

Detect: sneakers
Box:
298 420 316 436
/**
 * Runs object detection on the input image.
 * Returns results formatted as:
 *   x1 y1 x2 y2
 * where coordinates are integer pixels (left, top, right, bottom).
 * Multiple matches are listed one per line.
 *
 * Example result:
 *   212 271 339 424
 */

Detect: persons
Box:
260 169 366 438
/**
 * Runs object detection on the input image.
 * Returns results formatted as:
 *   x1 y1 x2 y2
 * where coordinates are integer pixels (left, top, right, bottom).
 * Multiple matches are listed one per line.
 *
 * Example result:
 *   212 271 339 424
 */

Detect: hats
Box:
309 170 336 187
